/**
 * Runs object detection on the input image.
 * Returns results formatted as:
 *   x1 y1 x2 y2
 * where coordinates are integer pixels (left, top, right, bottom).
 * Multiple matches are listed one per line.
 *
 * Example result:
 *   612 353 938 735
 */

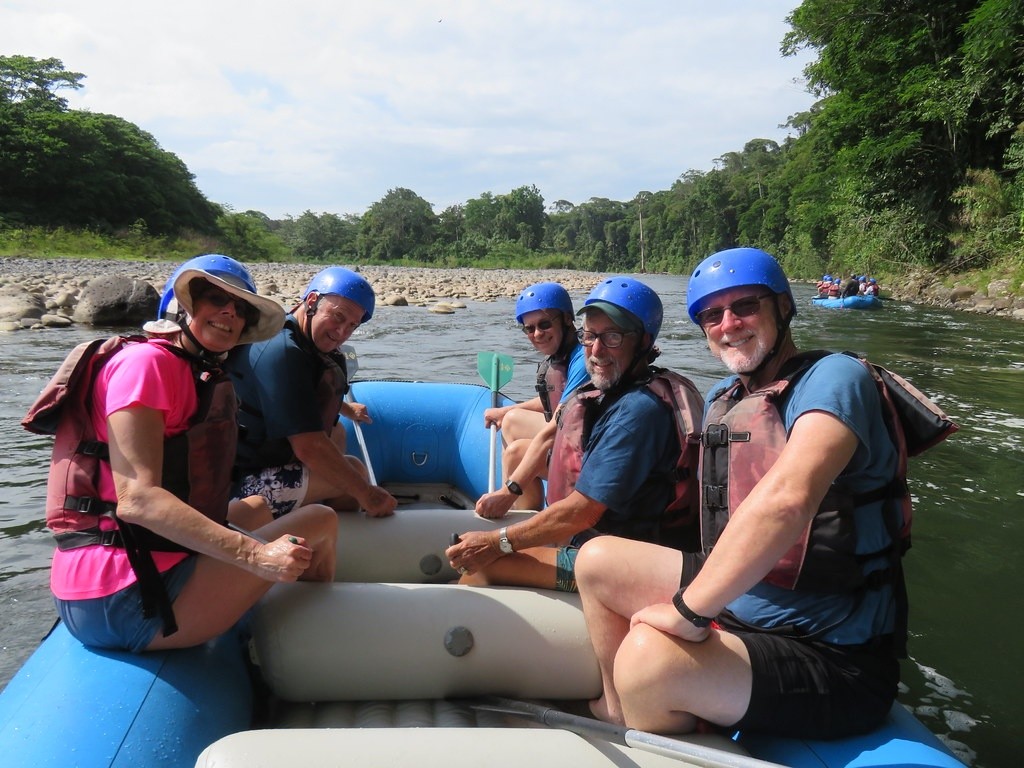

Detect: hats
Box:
141 268 286 362
576 300 643 332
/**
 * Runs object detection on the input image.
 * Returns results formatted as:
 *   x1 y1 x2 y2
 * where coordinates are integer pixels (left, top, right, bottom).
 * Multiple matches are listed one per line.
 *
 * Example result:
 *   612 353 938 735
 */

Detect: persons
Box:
817 274 879 299
20 256 338 651
573 248 956 740
476 283 591 519
236 266 398 518
444 276 706 589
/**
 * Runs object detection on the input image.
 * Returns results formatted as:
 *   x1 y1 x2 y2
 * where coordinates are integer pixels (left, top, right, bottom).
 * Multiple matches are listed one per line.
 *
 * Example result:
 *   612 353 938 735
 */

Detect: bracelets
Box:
672 587 713 627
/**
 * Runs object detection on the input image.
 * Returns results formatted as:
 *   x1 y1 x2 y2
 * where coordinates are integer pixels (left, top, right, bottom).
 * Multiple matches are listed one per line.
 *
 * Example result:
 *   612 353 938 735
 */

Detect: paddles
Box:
454 694 799 768
224 519 297 544
336 344 380 486
475 349 514 494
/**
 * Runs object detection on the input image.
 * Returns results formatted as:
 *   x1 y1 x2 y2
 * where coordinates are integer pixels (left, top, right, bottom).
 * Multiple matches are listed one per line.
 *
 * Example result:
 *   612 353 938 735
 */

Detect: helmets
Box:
515 282 576 324
870 278 876 285
302 267 375 324
851 274 856 278
158 254 258 319
827 277 833 281
585 277 663 345
823 275 830 281
859 276 866 281
835 278 841 284
686 248 797 325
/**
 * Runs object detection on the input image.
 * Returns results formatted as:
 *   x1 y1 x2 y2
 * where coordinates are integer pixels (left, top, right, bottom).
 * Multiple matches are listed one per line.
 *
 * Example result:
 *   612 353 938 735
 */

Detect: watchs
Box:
504 479 522 495
499 526 514 554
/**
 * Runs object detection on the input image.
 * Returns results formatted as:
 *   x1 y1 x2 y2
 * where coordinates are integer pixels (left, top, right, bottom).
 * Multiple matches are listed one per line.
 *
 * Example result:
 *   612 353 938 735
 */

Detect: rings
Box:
461 568 468 573
456 561 462 568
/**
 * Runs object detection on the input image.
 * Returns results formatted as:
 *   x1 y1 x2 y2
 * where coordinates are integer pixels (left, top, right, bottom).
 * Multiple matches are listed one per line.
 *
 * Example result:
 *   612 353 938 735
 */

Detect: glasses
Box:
575 327 635 348
522 313 561 335
201 285 258 320
695 293 775 327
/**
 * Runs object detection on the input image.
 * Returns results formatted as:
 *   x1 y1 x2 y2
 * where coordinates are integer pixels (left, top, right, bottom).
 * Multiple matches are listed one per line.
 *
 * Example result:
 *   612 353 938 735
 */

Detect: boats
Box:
813 294 884 313
